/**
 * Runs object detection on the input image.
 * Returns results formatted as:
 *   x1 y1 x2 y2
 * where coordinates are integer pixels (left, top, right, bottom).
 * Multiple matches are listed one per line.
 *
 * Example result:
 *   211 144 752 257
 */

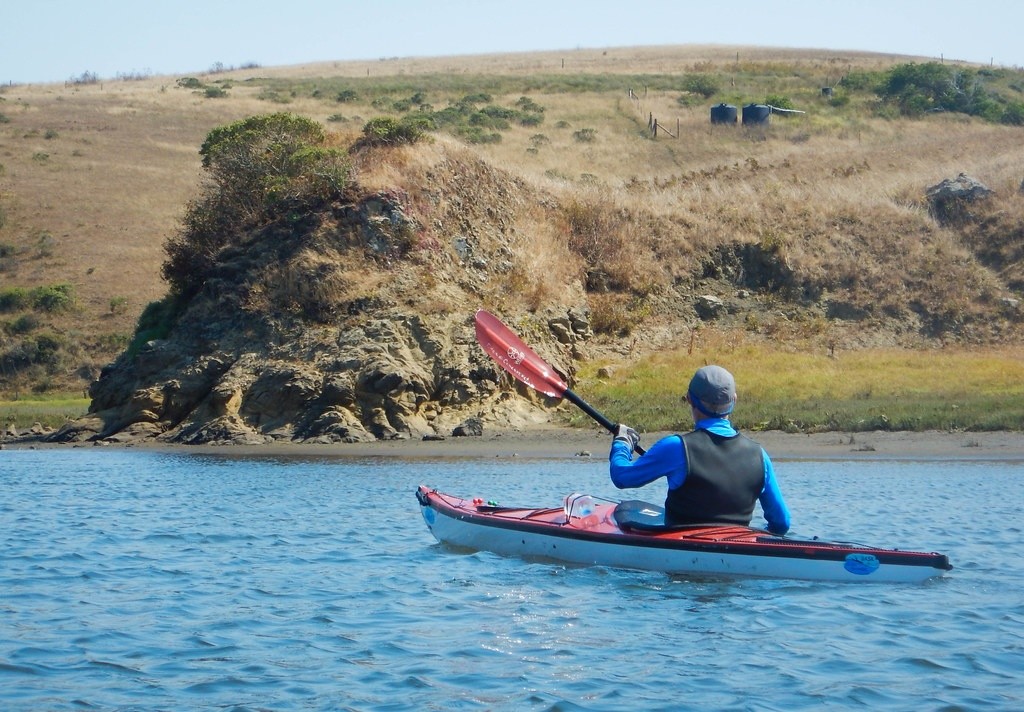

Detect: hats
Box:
688 365 735 418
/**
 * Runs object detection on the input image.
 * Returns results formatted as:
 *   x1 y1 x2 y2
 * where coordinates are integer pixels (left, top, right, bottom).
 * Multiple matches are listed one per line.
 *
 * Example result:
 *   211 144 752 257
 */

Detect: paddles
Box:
473 310 649 456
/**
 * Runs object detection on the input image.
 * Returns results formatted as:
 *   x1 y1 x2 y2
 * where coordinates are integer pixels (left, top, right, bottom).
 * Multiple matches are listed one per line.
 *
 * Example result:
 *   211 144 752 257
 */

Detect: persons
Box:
608 365 791 535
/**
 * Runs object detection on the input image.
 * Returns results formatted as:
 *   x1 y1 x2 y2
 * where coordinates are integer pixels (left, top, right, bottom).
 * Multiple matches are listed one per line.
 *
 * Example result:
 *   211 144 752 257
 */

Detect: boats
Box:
417 484 950 584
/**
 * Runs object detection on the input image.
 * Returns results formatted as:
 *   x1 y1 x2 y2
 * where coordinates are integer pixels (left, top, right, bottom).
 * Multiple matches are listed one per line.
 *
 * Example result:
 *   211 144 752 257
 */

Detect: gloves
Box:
613 423 640 454
